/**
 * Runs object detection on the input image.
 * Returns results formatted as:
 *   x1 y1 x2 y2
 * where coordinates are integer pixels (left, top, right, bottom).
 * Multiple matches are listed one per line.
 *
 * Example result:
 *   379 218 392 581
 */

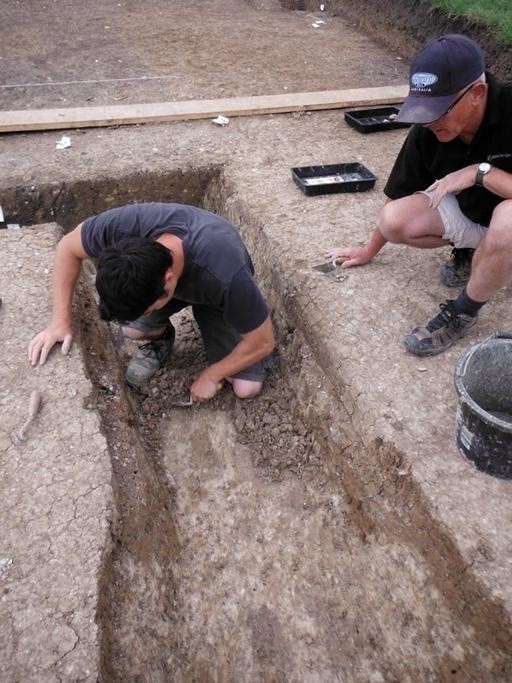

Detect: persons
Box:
323 32 512 357
25 203 275 402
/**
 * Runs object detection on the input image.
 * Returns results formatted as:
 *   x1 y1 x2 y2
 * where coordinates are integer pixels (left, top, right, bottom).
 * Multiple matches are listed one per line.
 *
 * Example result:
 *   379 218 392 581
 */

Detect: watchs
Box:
474 160 491 186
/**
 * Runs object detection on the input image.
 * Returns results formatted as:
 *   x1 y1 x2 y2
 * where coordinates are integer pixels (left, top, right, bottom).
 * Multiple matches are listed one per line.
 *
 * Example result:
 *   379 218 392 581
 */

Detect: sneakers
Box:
441 245 478 291
121 327 178 390
401 296 481 358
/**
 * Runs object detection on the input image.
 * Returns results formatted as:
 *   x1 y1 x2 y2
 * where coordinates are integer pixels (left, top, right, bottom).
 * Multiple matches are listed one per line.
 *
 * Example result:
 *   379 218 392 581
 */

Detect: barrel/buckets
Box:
453 331 512 482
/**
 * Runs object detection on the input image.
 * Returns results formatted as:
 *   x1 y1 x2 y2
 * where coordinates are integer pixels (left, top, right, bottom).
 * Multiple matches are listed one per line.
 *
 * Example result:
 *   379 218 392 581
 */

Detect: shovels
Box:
310 257 351 274
171 383 223 406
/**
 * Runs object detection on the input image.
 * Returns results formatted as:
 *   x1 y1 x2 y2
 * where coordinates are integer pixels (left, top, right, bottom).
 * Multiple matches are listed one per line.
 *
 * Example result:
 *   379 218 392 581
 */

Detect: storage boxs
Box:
290 159 377 196
341 105 412 135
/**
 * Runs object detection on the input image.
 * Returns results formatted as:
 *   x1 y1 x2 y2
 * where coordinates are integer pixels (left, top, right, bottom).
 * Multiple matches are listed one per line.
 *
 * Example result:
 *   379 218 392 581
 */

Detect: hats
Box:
390 33 489 128
